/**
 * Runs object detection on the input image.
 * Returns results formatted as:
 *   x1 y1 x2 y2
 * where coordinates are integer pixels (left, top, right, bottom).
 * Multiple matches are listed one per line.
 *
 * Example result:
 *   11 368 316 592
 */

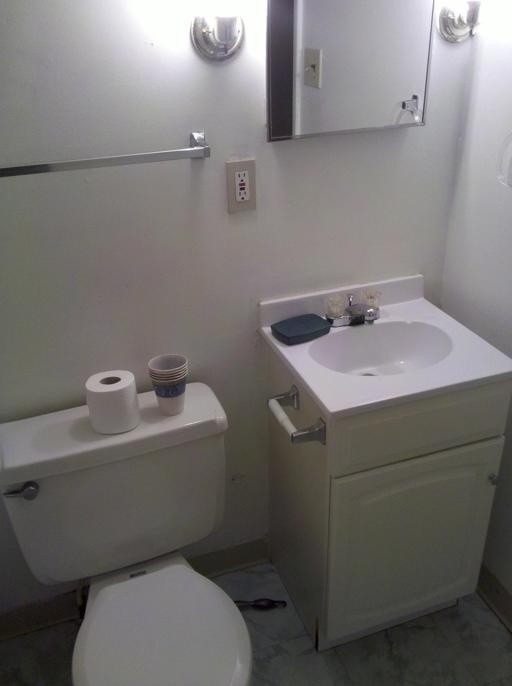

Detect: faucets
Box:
342 304 379 325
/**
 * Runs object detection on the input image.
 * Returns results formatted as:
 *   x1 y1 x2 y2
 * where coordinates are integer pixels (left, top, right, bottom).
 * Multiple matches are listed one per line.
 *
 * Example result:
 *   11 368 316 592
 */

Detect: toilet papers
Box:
85 369 142 436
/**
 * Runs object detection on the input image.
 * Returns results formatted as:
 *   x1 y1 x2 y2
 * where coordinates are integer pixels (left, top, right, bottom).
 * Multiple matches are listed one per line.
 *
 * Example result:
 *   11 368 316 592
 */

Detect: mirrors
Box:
266 0 434 144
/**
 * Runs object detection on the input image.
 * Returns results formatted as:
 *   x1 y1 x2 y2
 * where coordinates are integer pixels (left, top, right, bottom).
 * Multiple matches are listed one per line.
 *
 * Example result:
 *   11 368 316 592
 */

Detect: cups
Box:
149 354 190 413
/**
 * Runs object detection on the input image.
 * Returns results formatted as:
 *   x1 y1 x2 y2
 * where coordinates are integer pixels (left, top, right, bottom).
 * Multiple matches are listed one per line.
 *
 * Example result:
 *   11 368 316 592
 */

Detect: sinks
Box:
255 298 512 422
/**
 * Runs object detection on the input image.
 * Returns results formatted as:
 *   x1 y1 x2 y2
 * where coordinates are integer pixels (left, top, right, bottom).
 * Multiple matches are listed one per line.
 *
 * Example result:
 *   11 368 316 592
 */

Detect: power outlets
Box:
226 159 256 214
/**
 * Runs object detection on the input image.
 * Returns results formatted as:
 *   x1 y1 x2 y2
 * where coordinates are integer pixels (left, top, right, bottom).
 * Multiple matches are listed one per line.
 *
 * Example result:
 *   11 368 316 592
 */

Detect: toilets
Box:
0 381 254 686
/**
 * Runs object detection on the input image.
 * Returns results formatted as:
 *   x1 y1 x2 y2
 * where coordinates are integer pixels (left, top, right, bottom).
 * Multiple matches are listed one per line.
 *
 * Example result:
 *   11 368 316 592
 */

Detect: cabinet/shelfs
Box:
263 354 512 652
